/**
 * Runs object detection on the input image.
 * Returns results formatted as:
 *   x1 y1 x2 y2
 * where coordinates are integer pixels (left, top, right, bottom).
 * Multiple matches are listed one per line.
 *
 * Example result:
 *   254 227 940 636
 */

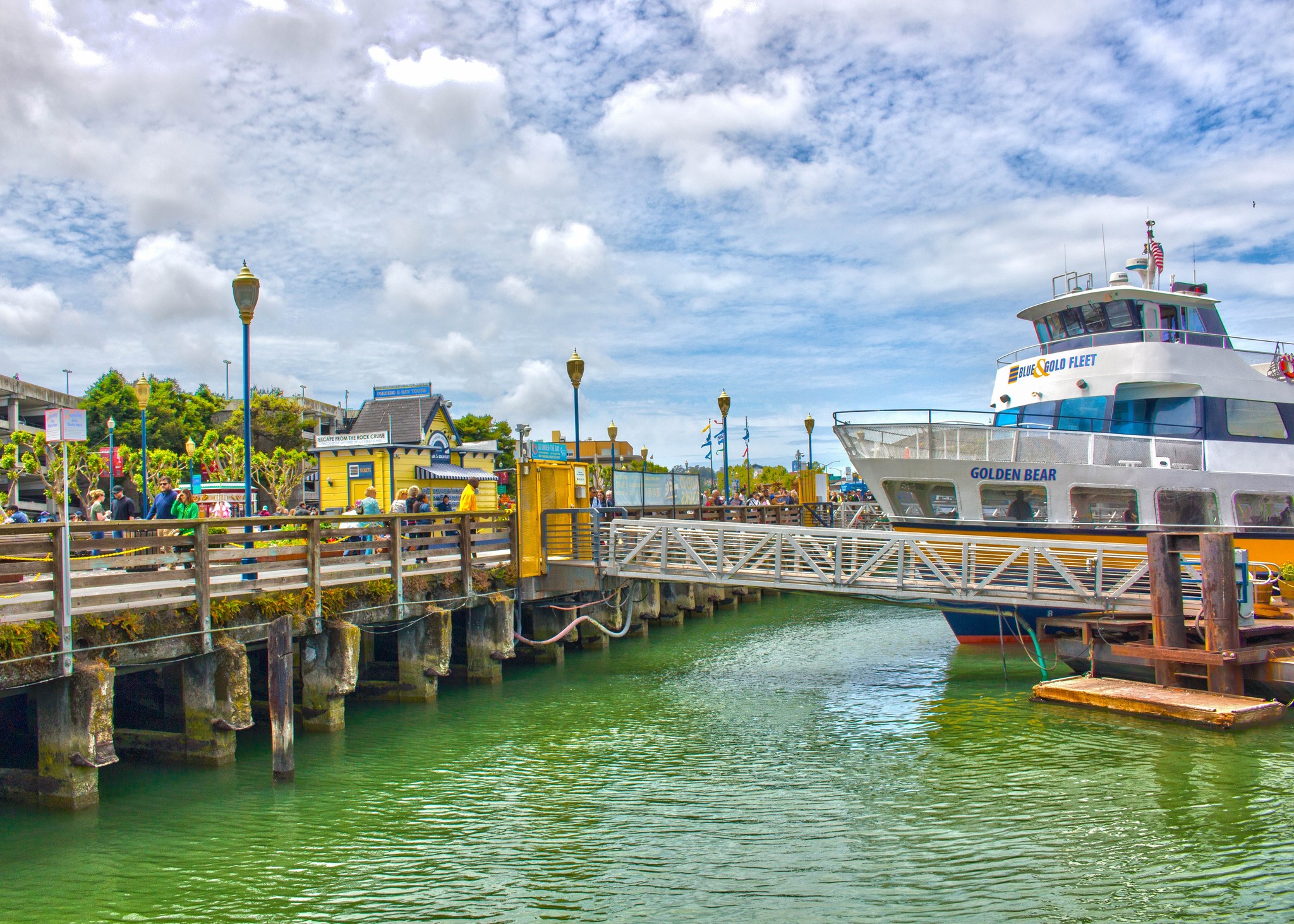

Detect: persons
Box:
439 495 460 547
589 487 799 561
1124 499 1138 530
343 486 390 567
389 488 407 560
6 504 28 523
1006 490 1032 527
258 501 319 532
90 489 112 571
413 492 432 564
38 511 111 523
402 485 419 560
171 488 199 580
145 476 179 570
110 485 135 569
828 489 875 515
459 477 488 566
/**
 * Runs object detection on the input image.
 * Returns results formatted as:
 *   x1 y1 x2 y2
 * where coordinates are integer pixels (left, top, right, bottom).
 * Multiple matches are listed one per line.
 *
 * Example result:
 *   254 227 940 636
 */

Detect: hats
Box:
263 505 268 510
111 485 123 493
41 511 50 516
857 489 862 491
74 511 82 515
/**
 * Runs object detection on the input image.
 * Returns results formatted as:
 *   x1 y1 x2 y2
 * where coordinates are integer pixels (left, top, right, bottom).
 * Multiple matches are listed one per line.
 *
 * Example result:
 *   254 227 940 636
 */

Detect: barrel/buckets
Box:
1252 583 1274 604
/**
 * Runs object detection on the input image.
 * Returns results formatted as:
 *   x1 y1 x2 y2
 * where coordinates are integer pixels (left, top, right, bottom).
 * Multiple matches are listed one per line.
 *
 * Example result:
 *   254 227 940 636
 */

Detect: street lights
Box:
829 467 842 485
804 412 815 469
61 368 73 395
812 459 840 473
685 459 688 473
134 372 152 517
619 455 624 469
606 420 618 469
222 360 231 400
231 258 261 580
640 444 649 471
565 347 585 462
106 415 116 519
852 470 857 493
300 385 306 397
185 435 196 496
716 388 732 520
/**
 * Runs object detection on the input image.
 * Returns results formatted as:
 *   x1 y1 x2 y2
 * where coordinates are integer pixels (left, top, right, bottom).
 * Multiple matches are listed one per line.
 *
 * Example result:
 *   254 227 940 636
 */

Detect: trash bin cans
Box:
122 529 161 573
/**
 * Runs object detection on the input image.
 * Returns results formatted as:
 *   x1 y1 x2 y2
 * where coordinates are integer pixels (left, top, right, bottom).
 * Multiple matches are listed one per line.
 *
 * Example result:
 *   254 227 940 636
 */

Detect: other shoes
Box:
93 568 107 571
415 560 422 563
471 563 486 566
366 563 380 566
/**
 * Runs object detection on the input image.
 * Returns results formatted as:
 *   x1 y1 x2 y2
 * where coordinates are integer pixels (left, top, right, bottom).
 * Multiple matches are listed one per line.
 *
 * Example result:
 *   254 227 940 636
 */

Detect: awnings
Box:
415 463 499 480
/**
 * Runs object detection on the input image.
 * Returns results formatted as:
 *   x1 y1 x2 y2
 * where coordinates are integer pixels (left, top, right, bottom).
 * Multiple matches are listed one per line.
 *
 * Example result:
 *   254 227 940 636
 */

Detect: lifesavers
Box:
1281 355 1294 378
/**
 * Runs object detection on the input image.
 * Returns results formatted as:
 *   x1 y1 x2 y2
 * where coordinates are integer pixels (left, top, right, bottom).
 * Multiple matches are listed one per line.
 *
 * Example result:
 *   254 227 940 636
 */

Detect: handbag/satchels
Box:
168 529 182 536
357 500 363 515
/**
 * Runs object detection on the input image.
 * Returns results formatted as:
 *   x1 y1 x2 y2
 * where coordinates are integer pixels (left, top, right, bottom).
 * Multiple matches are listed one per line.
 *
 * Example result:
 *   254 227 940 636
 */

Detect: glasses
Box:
114 492 120 494
180 494 185 497
590 491 596 493
159 483 169 486
102 495 105 497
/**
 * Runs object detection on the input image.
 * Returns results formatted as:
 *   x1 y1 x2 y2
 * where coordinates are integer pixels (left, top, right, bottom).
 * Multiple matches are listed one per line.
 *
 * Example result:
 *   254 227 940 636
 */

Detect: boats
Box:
829 211 1294 651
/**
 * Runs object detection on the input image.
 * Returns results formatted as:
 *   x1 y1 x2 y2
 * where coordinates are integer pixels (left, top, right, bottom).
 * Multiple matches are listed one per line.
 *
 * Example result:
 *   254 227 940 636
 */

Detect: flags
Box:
701 428 749 448
1151 240 1164 274
